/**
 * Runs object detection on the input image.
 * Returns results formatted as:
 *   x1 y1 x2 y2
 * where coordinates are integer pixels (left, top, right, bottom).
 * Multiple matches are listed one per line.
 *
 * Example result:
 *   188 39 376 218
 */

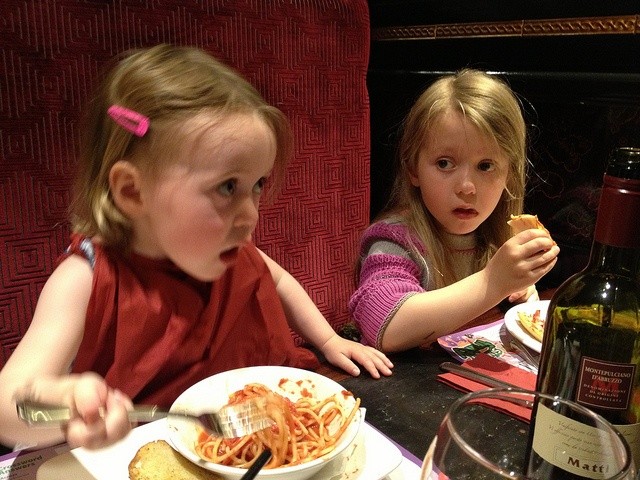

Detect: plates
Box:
503 301 552 352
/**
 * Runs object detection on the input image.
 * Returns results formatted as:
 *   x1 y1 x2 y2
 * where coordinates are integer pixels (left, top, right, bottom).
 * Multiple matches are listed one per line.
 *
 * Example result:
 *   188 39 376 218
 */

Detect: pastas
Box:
193 382 362 471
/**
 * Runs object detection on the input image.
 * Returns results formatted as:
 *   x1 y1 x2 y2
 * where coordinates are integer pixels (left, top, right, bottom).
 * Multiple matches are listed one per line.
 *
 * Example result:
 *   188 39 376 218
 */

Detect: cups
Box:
422 388 637 480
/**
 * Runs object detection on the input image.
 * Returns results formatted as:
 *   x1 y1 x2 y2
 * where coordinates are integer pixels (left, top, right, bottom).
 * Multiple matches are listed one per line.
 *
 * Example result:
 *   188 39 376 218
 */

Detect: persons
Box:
0 43 394 451
347 67 561 352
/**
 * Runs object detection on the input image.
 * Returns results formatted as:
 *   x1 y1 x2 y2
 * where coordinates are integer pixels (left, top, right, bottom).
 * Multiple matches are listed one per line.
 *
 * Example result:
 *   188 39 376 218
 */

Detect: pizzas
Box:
516 308 546 342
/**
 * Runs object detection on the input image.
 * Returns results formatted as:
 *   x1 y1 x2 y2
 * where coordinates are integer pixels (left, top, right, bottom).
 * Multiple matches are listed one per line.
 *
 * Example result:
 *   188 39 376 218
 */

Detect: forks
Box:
13 397 274 440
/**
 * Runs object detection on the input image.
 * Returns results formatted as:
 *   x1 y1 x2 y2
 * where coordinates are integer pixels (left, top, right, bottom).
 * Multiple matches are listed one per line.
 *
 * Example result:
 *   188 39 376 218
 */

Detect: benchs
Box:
1 0 371 385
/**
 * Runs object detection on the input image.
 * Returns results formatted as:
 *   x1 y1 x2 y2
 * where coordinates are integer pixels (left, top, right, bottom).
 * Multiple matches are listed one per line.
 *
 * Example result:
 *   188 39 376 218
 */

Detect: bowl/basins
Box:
164 366 360 480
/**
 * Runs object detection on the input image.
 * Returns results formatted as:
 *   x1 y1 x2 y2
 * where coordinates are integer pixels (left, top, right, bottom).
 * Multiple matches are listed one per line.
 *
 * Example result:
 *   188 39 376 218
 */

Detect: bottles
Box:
524 147 640 477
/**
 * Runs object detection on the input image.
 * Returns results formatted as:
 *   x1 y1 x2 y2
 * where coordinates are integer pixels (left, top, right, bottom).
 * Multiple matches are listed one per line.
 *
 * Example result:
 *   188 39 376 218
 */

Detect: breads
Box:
508 213 557 249
128 438 223 479
